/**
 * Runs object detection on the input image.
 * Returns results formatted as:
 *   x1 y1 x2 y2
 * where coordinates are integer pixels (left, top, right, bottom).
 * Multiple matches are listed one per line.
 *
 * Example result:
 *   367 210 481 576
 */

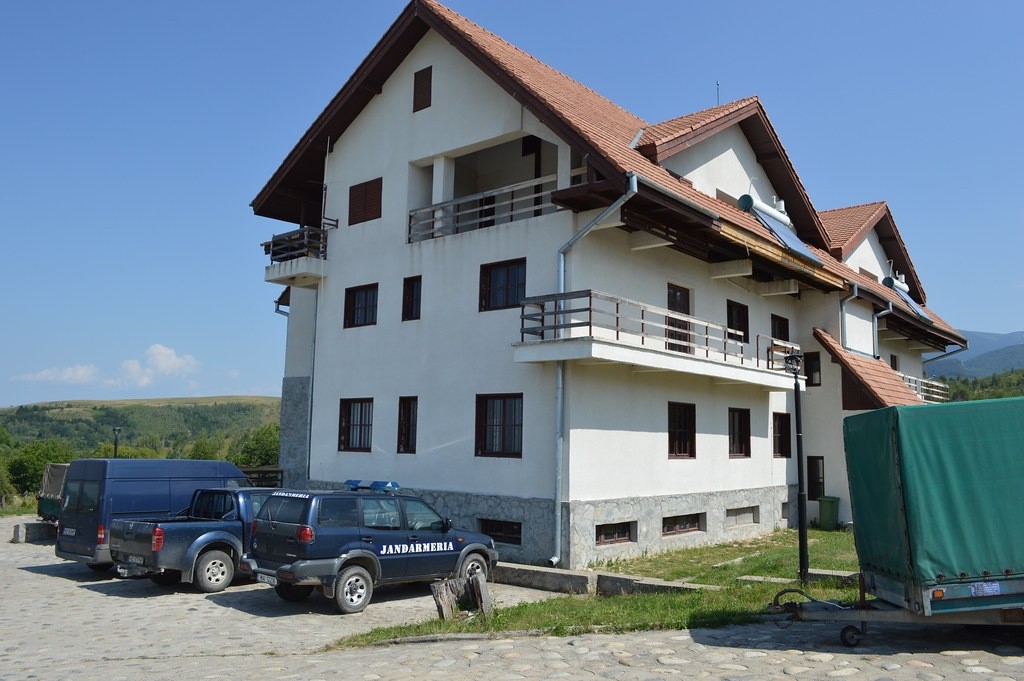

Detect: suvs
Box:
240 480 500 614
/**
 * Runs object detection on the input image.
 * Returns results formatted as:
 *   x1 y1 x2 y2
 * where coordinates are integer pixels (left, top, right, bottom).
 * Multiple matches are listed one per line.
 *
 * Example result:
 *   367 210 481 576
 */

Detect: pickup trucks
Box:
105 486 291 595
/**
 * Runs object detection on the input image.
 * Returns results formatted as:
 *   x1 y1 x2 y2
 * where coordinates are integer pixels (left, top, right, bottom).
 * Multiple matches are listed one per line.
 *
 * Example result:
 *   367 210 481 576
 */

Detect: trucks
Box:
35 462 71 528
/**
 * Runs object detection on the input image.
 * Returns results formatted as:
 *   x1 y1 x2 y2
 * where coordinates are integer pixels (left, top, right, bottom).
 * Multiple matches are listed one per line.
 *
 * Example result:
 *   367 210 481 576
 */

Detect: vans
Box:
54 457 267 572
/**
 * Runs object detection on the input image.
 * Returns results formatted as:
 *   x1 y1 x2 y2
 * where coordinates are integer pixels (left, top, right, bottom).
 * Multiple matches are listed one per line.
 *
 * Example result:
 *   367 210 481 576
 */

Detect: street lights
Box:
112 426 122 458
782 352 813 589
